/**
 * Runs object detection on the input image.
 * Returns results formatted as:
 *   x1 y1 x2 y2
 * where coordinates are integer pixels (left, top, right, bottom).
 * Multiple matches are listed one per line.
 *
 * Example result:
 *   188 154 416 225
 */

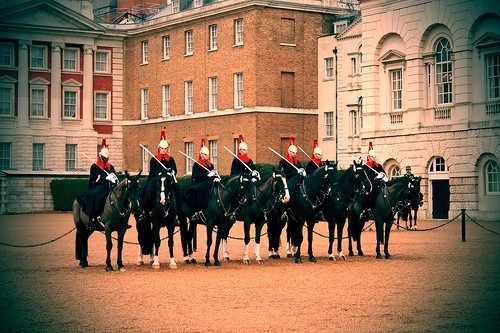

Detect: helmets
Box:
312 138 322 155
288 136 297 154
367 142 376 158
238 134 248 151
99 137 109 159
199 139 209 155
158 129 168 149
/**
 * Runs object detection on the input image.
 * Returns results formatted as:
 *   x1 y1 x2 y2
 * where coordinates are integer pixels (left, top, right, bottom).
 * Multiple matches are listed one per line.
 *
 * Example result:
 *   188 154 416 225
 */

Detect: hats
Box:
406 166 411 170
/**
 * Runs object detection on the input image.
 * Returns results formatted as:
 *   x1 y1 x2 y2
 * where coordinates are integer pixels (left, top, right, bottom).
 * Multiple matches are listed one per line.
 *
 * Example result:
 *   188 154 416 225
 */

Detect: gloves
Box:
215 178 220 183
382 177 389 183
207 172 216 177
106 173 117 181
377 173 384 178
252 172 257 177
298 170 303 173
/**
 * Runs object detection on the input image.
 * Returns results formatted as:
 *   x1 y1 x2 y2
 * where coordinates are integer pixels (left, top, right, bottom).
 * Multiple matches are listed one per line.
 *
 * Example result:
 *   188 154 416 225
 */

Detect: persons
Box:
88 147 132 229
278 145 306 222
305 146 324 174
360 149 386 220
138 140 180 226
230 141 261 222
404 166 423 207
191 146 220 223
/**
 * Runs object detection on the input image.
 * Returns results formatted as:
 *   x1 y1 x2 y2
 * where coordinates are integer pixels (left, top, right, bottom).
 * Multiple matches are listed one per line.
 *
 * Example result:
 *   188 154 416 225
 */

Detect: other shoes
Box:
127 224 132 229
89 222 93 231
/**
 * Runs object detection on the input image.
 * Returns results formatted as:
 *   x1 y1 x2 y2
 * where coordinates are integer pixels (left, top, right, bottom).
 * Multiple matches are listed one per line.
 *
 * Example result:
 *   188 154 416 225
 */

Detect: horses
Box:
222 167 291 265
72 170 142 271
178 172 255 266
348 175 423 258
285 160 373 260
266 159 339 263
137 167 180 269
